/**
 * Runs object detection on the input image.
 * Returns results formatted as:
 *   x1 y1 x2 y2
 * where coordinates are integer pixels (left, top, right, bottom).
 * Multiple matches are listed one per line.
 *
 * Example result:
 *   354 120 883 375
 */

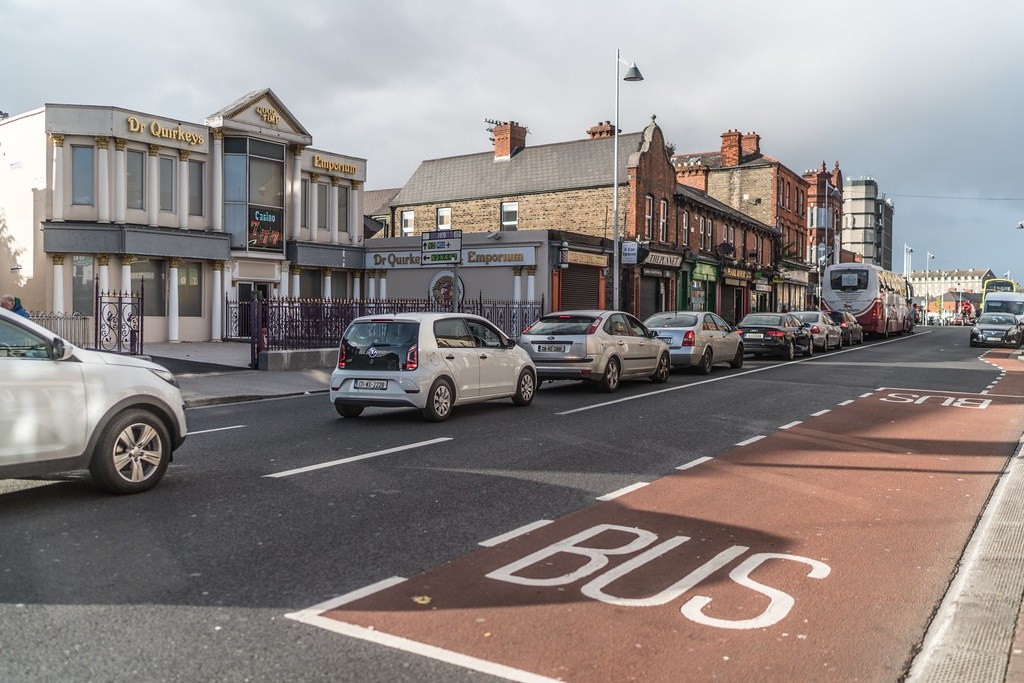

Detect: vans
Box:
983 292 1024 332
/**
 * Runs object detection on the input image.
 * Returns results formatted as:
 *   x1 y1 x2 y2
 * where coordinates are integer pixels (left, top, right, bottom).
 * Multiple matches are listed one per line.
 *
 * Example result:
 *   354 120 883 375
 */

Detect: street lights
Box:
925 250 935 326
612 47 643 310
1004 270 1010 280
903 242 913 280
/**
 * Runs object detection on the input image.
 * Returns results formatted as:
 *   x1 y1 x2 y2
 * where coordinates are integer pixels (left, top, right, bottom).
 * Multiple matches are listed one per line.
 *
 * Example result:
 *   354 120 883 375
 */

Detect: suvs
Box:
1 307 190 489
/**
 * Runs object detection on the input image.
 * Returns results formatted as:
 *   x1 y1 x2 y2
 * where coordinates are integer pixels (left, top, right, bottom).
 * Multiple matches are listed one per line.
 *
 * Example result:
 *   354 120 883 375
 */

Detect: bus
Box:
821 262 915 338
981 278 1020 314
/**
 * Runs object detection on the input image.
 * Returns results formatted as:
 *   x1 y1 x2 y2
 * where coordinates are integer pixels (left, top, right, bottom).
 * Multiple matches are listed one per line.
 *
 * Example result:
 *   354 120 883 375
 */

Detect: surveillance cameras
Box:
486 230 501 241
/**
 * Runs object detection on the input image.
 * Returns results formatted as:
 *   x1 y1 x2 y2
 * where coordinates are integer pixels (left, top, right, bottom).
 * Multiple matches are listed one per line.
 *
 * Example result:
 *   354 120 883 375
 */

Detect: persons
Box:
0 295 30 320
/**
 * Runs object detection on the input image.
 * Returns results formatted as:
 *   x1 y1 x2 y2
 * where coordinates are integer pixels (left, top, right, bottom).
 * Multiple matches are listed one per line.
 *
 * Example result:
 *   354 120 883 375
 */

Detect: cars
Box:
328 311 539 419
518 309 672 392
734 314 813 362
824 311 864 347
639 310 743 373
784 311 844 353
969 312 1024 348
